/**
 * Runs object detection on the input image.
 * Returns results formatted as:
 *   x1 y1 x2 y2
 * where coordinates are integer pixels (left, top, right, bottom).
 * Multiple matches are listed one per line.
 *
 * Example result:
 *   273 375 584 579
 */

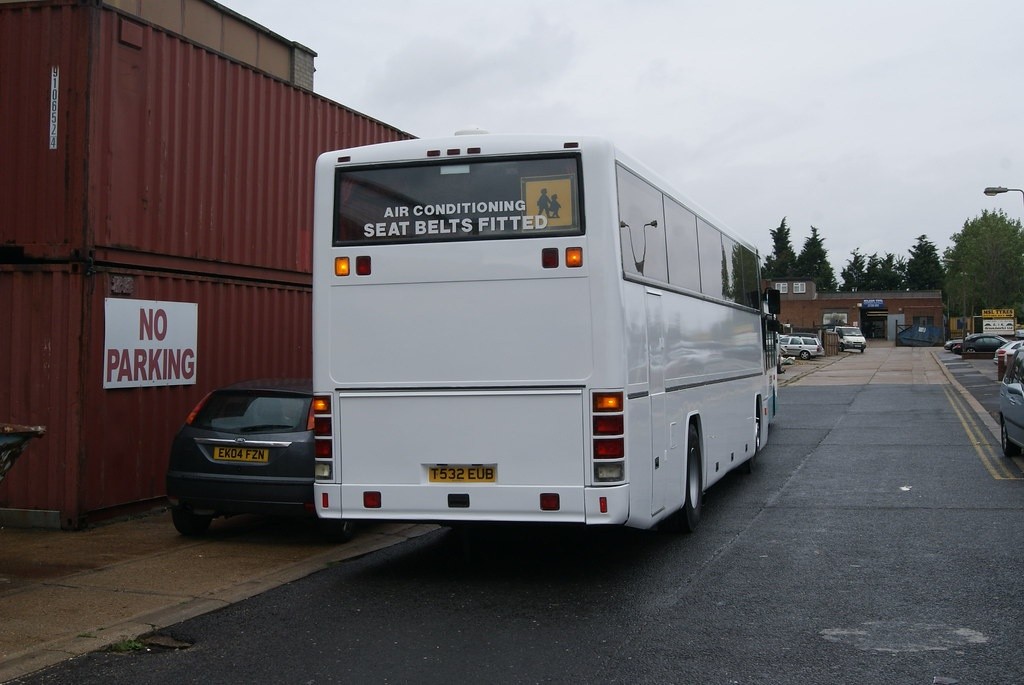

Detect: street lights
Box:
984 187 1024 204
940 258 967 360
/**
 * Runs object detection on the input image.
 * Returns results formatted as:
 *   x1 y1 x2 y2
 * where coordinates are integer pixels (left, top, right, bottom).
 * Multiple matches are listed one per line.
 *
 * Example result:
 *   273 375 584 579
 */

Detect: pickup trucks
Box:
826 326 866 353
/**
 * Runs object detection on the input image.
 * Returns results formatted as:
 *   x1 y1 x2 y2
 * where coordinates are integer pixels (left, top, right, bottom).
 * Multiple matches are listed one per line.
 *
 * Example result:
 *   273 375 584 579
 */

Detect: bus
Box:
314 131 790 528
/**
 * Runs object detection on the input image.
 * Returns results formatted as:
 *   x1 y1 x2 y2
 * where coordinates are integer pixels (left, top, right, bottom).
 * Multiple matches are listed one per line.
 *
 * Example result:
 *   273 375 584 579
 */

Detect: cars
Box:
1000 347 1024 459
165 382 357 544
779 336 825 360
994 340 1024 365
945 334 1009 354
